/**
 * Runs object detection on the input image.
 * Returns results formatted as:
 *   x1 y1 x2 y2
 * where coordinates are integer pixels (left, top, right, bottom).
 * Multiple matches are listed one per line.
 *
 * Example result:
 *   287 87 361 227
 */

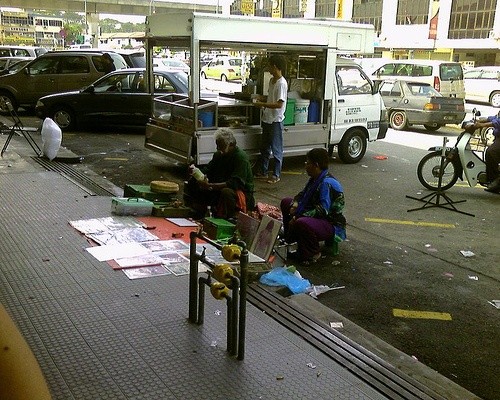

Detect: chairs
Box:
0 95 42 157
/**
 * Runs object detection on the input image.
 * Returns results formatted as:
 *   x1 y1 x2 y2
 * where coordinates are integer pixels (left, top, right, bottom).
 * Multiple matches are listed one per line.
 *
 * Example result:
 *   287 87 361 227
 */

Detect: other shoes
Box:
487 177 500 188
265 175 280 184
302 257 322 267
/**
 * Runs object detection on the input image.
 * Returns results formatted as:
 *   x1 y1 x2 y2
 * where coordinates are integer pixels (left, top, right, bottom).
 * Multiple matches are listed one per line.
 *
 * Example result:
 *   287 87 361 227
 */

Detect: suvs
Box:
0 50 133 115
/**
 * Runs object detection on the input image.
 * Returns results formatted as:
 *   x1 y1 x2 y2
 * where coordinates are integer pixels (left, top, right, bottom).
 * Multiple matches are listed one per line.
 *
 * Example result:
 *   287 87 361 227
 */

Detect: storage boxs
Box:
283 98 295 125
111 184 190 217
203 217 236 240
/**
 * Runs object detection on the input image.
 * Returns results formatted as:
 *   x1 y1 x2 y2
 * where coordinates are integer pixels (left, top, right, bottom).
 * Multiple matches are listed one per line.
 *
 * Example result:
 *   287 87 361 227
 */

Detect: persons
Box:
102 53 116 75
280 148 347 266
188 128 256 218
251 55 289 184
464 110 500 195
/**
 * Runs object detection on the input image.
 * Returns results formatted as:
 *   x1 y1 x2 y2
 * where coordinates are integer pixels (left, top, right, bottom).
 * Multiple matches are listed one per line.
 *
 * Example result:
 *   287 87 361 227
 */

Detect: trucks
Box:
143 11 389 176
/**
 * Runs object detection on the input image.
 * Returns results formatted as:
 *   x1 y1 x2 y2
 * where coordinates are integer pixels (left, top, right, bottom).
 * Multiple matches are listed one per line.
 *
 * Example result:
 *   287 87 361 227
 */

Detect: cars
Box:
34 67 190 131
463 66 500 108
201 54 243 82
380 80 466 131
0 45 190 76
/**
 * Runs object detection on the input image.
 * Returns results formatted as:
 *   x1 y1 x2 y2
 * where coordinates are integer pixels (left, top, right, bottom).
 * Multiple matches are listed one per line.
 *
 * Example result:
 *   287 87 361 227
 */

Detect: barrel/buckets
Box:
282 98 296 125
294 99 310 124
308 101 319 122
199 111 213 127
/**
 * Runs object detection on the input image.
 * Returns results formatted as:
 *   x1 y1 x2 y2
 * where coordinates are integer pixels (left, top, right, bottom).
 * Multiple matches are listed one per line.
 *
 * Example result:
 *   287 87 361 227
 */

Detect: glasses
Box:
305 160 320 165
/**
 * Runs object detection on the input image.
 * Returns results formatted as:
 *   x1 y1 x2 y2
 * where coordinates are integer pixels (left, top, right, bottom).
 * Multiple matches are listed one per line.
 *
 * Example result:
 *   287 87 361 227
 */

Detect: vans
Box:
368 60 466 104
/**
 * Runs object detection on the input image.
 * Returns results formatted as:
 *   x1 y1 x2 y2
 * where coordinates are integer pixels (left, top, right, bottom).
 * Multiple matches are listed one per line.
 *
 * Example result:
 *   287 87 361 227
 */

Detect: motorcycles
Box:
417 108 500 191
